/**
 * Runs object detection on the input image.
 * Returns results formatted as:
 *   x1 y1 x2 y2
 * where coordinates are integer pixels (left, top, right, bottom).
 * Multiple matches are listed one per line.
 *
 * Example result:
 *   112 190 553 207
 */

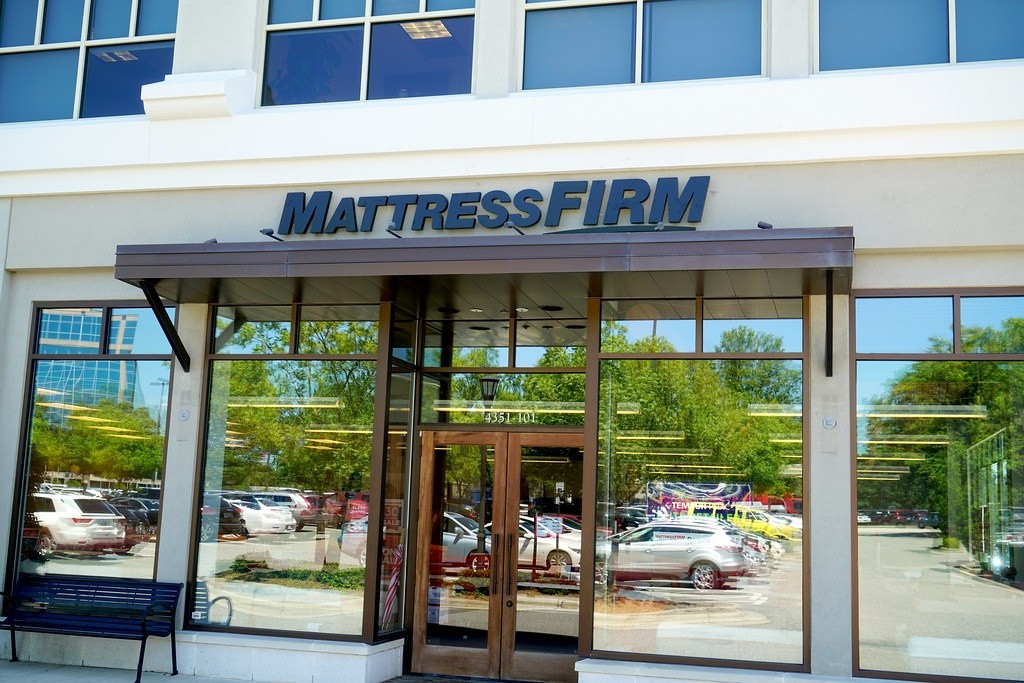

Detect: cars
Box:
24 482 370 559
997 505 1024 527
856 510 943 530
338 503 614 586
595 504 803 591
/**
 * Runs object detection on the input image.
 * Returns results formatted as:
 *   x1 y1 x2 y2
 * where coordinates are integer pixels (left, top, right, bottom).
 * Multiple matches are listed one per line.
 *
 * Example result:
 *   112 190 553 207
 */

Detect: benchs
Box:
0 572 184 683
189 581 234 627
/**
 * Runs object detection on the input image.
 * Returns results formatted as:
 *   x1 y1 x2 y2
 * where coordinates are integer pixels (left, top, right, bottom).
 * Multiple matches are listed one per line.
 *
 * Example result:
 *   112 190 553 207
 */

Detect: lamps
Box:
259 228 283 241
205 238 217 243
386 222 402 238
655 221 665 232
505 222 526 235
758 221 773 229
